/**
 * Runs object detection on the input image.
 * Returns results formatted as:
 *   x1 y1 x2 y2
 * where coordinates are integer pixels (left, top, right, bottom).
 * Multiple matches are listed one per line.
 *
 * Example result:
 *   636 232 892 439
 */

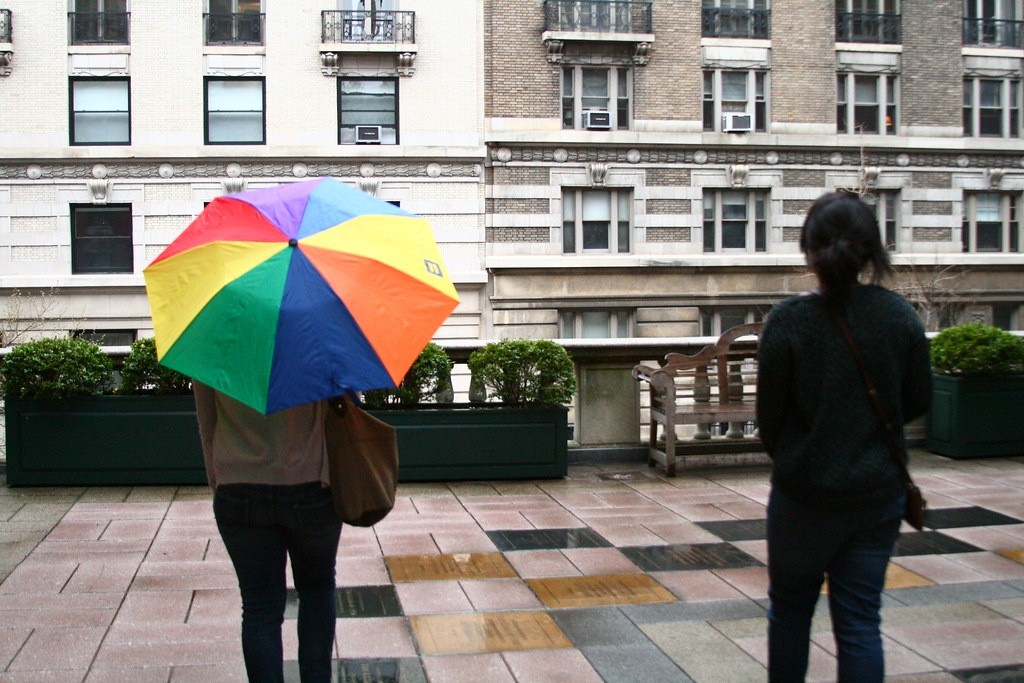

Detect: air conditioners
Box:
355 125 382 144
722 112 752 132
584 111 612 131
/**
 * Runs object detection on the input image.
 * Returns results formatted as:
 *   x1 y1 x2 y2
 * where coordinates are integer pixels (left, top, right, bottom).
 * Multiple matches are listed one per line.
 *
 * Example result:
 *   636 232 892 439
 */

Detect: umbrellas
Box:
142 176 461 414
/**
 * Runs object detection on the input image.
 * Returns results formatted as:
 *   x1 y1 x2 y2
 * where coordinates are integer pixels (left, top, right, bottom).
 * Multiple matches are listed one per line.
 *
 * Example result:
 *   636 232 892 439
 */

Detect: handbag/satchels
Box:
323 389 398 527
900 467 925 532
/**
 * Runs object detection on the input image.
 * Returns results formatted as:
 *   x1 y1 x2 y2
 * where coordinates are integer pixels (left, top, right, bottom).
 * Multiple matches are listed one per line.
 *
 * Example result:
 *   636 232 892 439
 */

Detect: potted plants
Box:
925 322 1024 461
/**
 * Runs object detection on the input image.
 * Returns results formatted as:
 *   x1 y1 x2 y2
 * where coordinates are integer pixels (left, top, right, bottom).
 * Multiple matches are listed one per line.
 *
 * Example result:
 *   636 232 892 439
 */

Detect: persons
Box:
758 188 935 683
193 376 342 683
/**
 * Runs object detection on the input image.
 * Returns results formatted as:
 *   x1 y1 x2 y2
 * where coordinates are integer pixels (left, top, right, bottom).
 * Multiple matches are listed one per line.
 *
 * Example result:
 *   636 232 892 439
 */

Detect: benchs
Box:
631 321 768 479
